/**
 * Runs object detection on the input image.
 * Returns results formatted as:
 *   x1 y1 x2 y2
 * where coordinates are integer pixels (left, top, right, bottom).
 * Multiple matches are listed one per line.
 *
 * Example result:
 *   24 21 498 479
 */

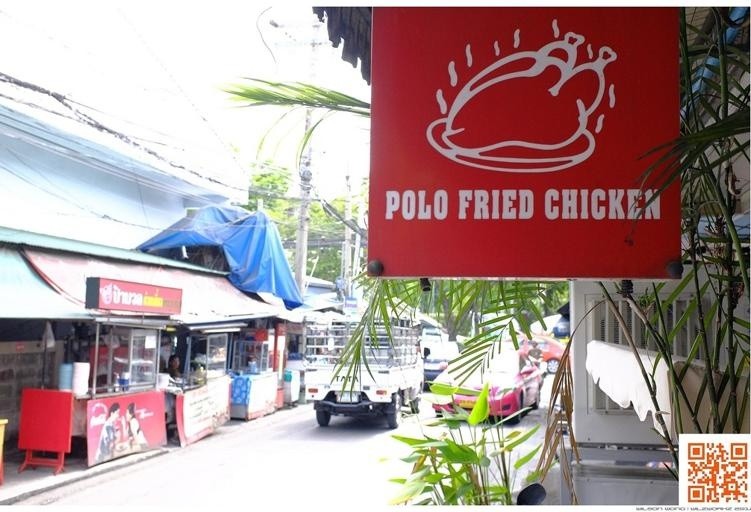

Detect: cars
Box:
421 312 570 425
209 345 225 369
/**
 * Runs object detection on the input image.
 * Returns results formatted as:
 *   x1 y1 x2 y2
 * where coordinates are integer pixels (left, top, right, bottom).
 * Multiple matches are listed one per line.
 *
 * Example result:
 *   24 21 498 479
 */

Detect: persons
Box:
95 402 121 462
165 353 182 380
122 402 148 449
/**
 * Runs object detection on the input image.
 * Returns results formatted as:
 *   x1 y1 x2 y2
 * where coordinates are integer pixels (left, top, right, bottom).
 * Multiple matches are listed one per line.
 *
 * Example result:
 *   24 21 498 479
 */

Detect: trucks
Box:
303 311 424 429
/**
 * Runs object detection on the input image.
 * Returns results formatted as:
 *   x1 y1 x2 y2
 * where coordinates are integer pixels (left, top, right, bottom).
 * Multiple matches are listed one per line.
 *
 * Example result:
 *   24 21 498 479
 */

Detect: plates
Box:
59 362 90 395
